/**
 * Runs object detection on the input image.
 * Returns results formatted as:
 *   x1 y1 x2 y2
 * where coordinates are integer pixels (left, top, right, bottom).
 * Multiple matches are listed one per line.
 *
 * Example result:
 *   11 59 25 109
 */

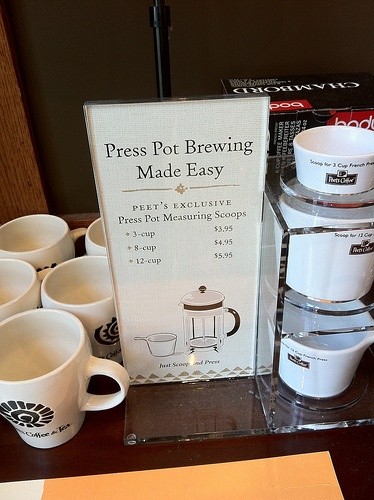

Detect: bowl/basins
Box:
293 125 374 194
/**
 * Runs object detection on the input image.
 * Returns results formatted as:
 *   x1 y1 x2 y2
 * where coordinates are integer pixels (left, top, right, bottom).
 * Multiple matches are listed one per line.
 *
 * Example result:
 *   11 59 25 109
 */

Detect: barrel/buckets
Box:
275 185 373 303
267 288 374 397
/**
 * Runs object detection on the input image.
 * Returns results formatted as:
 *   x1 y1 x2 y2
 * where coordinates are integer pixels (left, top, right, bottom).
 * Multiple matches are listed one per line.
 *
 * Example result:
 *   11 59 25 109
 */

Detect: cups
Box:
41 255 122 364
0 259 54 322
85 217 107 257
0 214 87 272
0 308 129 449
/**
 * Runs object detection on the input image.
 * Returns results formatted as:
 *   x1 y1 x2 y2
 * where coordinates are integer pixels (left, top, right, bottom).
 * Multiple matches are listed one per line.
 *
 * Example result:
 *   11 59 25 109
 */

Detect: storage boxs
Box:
220 73 374 187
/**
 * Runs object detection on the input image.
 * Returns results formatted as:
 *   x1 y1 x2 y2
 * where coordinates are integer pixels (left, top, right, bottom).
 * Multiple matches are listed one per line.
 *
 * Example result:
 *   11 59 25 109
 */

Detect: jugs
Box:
177 286 241 354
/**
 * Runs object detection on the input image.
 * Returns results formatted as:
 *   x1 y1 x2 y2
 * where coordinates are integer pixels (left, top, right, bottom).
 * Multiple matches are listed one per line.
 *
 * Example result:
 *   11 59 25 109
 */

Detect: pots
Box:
134 333 177 356
